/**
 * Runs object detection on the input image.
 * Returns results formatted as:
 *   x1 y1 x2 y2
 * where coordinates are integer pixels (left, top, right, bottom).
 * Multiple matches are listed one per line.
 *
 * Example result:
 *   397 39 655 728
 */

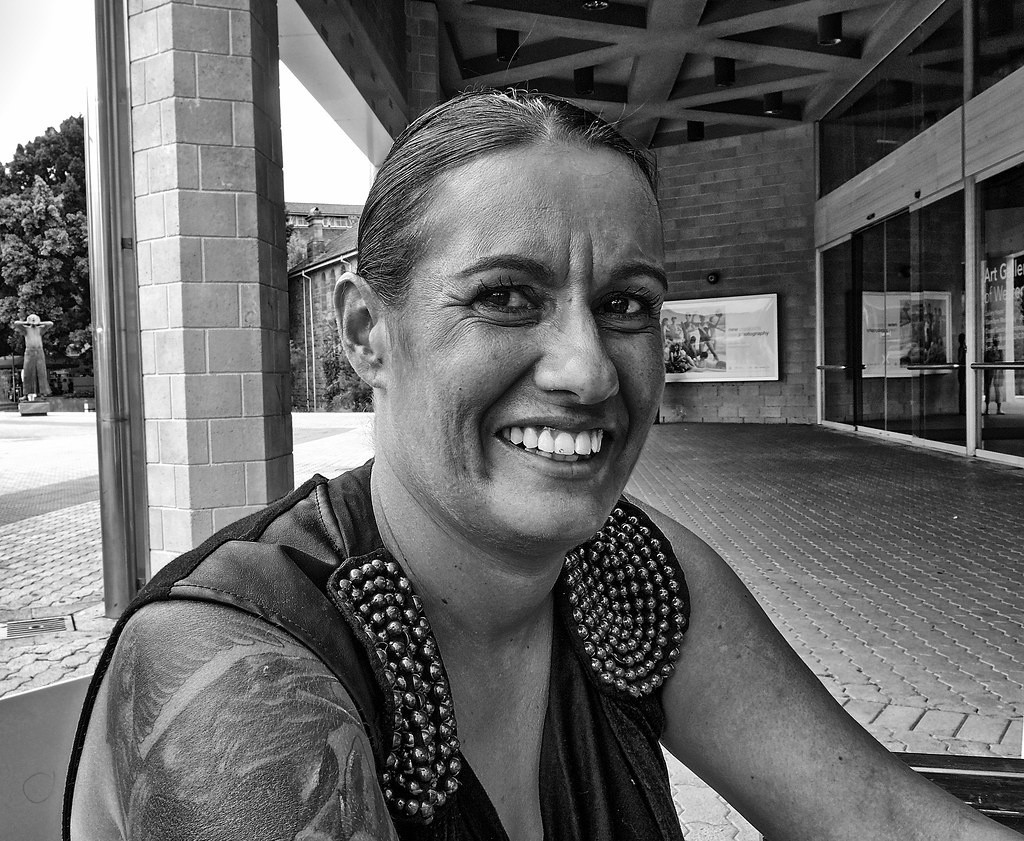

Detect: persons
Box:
55 83 1024 841
957 333 966 416
900 302 947 365
661 312 726 374
13 314 53 398
982 333 1005 416
